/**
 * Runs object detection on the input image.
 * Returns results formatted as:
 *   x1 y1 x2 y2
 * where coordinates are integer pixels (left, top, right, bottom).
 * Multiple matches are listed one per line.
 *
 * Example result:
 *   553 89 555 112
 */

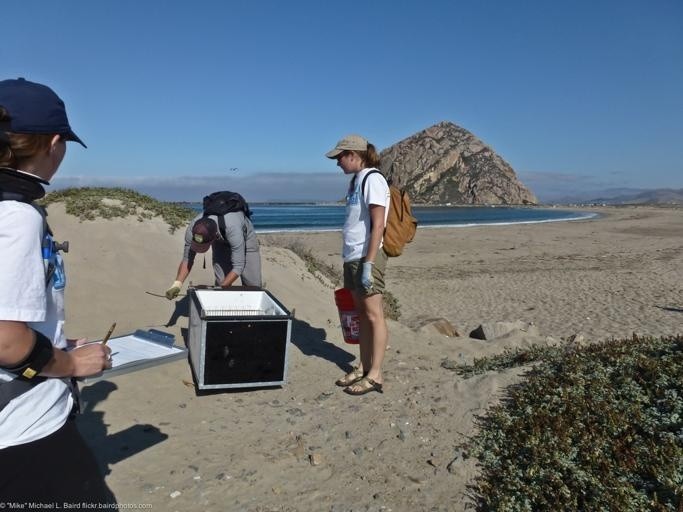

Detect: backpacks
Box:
362 169 418 257
202 190 253 240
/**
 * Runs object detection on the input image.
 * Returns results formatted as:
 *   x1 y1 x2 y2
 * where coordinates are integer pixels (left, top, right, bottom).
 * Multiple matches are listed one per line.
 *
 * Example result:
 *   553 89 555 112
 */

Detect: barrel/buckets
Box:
334 288 361 344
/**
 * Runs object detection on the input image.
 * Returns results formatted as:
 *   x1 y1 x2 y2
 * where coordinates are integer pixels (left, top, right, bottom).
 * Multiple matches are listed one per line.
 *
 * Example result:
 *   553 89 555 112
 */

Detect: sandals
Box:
335 368 383 394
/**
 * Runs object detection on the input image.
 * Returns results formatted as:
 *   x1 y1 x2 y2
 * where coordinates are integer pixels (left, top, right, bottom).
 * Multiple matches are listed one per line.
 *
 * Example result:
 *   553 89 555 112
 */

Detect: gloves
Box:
360 262 375 290
165 280 183 300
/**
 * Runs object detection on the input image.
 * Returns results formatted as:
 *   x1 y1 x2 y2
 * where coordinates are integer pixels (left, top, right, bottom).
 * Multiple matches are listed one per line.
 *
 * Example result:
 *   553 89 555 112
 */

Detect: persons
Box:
324 136 417 396
0 76 118 512
165 190 262 301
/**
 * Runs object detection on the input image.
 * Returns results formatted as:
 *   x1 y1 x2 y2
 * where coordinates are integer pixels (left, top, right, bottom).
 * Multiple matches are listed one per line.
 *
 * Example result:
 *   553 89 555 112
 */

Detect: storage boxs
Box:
185 285 293 397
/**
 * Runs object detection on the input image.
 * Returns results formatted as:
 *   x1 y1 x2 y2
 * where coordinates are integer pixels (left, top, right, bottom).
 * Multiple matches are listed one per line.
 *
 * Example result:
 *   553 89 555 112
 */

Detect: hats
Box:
191 218 217 253
0 77 88 148
325 134 367 159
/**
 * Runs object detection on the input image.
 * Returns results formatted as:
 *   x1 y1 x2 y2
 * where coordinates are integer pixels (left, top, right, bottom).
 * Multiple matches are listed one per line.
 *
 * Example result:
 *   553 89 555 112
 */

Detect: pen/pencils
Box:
101 322 116 345
